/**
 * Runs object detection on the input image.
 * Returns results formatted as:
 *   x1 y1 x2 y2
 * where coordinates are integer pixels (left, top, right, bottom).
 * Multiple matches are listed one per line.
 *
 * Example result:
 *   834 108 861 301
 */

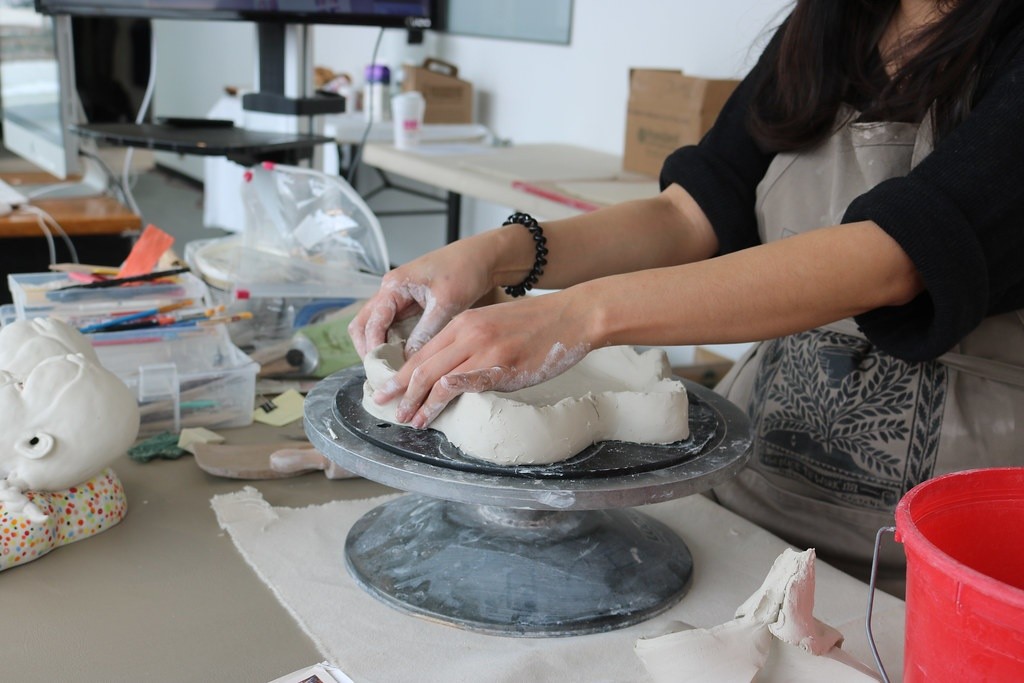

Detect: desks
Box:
204 95 493 272
362 139 663 222
0 170 141 306
67 123 335 166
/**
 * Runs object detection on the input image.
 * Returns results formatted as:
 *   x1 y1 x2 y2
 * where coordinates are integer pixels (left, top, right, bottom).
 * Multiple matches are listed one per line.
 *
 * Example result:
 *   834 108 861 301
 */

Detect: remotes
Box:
159 115 234 129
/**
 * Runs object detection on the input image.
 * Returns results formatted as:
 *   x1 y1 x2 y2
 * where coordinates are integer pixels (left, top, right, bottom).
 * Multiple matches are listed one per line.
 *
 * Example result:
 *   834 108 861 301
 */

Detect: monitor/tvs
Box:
35 0 440 30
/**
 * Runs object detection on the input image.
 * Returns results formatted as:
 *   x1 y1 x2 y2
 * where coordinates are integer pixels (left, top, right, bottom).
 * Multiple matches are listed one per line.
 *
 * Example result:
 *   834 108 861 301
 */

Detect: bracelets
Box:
501 210 549 298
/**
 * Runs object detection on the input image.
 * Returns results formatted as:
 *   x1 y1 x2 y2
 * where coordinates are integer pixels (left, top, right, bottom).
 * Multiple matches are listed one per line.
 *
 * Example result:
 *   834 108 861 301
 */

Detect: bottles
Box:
285 304 365 378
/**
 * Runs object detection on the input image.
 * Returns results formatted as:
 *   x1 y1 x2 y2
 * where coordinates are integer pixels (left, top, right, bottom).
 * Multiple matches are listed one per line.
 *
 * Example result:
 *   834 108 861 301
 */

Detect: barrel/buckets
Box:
865 466 1024 683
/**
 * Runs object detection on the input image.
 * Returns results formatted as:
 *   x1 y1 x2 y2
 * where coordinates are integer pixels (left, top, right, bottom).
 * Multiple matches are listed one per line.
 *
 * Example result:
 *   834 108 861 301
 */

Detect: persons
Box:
347 0 1024 431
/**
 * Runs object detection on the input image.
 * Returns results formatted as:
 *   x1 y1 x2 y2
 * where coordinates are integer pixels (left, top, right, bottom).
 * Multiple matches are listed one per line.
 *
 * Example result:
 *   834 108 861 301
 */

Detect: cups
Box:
391 90 426 151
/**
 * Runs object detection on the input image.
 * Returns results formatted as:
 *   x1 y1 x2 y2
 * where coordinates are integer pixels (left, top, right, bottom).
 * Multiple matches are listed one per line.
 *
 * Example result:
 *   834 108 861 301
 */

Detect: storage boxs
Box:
93 334 261 436
6 272 206 325
622 67 741 179
400 64 472 126
183 238 378 342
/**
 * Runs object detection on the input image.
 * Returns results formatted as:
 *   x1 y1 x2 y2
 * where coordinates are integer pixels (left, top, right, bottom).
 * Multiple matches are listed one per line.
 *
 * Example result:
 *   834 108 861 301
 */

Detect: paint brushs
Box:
46 264 252 335
